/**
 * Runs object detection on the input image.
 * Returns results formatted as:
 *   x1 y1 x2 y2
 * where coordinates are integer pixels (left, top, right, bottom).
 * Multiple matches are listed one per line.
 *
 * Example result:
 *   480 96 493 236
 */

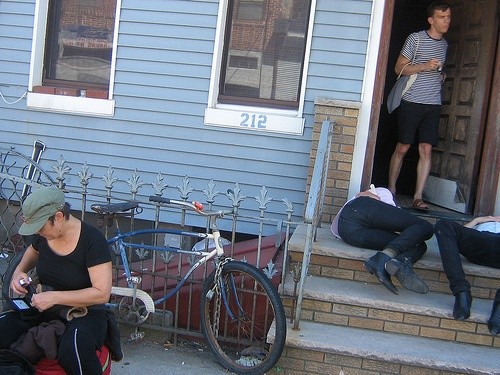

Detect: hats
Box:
18 185 65 235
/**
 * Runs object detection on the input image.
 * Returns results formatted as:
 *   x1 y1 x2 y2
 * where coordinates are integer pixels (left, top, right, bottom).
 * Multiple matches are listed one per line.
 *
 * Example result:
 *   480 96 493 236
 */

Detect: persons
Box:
330 184 434 296
388 1 451 210
0 185 114 375
435 215 500 336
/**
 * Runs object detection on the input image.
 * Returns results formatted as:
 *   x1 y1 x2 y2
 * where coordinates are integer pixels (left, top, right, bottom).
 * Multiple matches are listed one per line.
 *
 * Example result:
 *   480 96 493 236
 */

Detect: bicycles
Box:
2 188 288 375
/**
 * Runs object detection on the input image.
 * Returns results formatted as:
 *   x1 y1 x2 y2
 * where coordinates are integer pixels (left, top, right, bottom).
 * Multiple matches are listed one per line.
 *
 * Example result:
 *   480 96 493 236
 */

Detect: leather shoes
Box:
450 276 472 321
487 289 500 335
385 253 429 294
363 252 399 295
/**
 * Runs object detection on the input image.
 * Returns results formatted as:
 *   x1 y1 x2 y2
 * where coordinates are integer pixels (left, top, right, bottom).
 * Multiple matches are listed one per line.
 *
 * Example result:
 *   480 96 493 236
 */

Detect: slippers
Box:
412 199 429 209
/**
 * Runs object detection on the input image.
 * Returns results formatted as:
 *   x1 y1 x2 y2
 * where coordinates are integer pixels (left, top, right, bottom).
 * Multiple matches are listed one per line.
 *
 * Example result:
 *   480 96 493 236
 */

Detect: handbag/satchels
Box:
387 73 418 113
